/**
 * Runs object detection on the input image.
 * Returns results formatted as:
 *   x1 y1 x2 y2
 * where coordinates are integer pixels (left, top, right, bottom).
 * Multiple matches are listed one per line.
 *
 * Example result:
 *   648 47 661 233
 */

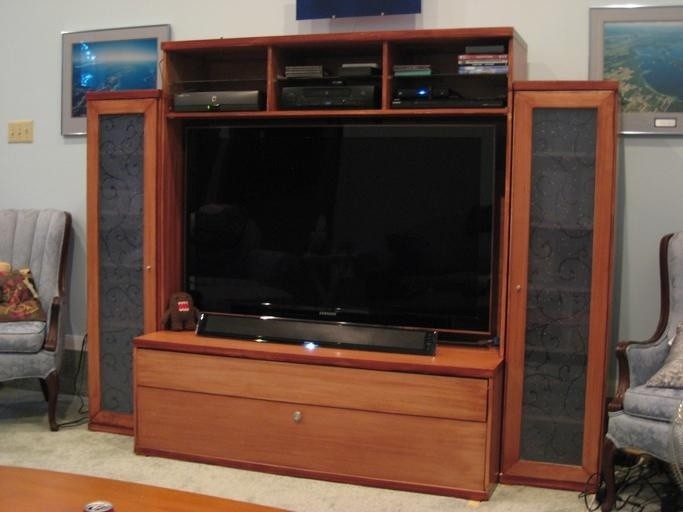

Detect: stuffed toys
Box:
158 291 201 331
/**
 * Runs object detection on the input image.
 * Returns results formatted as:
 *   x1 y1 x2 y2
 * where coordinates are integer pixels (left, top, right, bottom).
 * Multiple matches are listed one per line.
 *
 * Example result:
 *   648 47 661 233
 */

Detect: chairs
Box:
606 229 683 512
0 209 74 432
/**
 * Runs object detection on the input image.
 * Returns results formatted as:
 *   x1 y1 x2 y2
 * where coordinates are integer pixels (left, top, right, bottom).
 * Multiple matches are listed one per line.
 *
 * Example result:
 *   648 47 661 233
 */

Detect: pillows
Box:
645 325 683 388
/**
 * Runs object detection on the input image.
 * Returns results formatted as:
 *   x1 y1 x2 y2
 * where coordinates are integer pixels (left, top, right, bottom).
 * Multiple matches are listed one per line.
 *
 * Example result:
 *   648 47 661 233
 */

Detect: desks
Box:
0 465 295 512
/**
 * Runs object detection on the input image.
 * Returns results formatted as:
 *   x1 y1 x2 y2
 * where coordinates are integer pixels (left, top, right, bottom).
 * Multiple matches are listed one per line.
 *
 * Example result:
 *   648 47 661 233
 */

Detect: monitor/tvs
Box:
179 123 502 357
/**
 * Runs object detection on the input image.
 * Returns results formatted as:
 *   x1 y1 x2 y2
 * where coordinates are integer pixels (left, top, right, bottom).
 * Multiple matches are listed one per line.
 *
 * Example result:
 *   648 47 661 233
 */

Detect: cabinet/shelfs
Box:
86 26 620 502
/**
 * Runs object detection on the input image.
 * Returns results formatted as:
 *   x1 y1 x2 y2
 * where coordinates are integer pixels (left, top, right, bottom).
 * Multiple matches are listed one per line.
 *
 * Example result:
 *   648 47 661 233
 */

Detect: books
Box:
284 63 380 78
393 65 431 71
456 53 508 76
395 72 434 76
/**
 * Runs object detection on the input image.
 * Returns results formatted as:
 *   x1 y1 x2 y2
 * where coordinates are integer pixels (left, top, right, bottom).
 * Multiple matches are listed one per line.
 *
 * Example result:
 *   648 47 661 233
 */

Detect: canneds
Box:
83 500 114 512
